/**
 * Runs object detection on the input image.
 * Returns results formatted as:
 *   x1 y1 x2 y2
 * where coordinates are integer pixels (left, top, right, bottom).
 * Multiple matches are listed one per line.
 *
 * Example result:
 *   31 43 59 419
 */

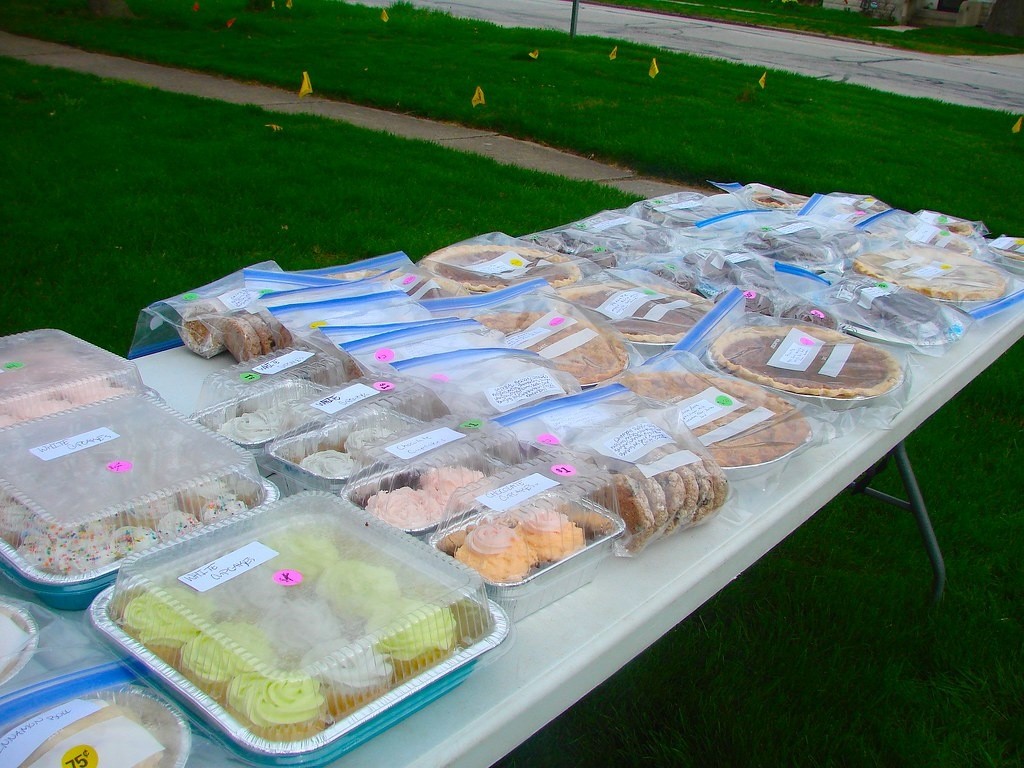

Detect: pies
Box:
417 186 1024 477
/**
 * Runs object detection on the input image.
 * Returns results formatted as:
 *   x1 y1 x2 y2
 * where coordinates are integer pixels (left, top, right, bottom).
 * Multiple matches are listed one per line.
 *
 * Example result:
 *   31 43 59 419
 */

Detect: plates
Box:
933 272 1015 304
718 427 816 481
707 348 906 412
843 312 964 348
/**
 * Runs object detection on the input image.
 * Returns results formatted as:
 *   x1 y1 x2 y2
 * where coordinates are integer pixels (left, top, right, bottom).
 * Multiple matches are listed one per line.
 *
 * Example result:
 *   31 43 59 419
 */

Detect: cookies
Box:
183 290 731 551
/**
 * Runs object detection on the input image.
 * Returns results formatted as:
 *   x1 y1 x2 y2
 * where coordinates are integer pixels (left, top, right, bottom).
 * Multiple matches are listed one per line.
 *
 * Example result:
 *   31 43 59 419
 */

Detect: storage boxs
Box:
85 489 516 768
0 390 280 613
339 416 524 545
429 448 627 623
256 371 452 499
190 346 348 458
0 325 166 429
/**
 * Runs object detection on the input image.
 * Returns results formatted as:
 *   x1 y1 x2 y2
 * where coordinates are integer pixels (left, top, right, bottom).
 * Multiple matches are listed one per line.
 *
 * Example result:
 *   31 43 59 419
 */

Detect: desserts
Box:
0 337 590 768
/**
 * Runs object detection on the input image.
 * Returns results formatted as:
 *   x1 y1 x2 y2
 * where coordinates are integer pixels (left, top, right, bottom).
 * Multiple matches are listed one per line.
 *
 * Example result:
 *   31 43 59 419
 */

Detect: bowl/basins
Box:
0 325 677 768
988 250 1024 275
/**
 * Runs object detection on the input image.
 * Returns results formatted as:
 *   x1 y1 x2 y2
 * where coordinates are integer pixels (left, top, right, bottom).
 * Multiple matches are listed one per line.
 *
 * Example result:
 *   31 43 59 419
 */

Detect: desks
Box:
0 193 1024 768
